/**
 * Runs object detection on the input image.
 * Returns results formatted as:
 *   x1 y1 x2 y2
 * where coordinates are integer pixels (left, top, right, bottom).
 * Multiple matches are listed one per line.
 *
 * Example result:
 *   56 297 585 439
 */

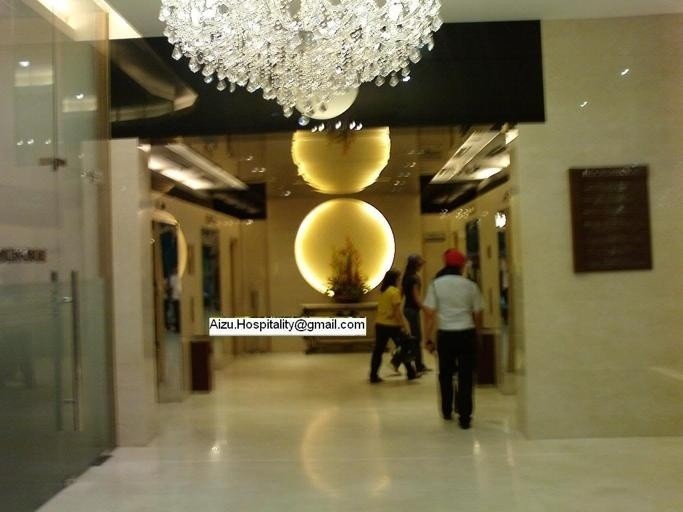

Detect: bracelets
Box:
424 340 432 347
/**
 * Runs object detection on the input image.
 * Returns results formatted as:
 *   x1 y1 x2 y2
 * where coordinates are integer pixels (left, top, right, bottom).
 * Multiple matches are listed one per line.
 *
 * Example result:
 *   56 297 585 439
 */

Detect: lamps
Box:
155 0 442 130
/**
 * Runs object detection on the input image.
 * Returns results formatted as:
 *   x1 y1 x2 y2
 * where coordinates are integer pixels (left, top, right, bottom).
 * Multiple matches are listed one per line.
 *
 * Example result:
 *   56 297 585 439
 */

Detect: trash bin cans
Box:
476 326 496 385
190 334 212 391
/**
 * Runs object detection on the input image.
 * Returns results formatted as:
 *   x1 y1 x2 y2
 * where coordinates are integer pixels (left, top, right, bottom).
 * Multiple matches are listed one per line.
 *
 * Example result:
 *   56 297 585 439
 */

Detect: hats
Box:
442 248 466 268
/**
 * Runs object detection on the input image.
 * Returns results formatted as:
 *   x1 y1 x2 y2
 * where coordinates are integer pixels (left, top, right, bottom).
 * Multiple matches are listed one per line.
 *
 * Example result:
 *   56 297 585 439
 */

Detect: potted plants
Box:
324 234 370 303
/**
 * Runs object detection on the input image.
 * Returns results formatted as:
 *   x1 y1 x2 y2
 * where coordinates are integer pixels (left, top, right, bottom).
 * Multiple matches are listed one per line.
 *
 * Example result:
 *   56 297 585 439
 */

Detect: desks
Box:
301 302 391 354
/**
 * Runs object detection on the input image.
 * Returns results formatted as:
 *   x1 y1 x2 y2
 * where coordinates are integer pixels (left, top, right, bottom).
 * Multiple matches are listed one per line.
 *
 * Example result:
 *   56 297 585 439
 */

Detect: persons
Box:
422 248 487 429
369 270 415 383
384 254 428 376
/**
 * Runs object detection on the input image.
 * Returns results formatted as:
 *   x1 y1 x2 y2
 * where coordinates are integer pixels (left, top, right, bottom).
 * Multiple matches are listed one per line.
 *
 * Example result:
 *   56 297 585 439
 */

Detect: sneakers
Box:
385 361 434 381
441 410 472 429
